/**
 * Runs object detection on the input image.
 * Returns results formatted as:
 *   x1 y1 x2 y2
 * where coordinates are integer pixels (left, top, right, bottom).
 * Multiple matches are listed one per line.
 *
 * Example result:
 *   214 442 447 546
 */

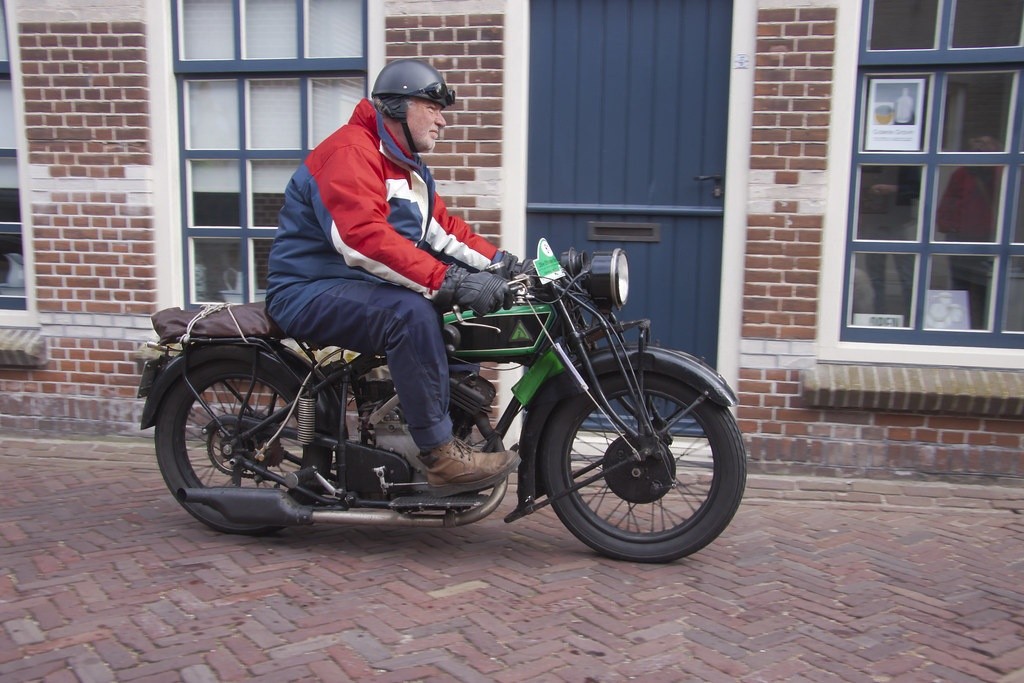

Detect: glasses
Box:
402 82 456 107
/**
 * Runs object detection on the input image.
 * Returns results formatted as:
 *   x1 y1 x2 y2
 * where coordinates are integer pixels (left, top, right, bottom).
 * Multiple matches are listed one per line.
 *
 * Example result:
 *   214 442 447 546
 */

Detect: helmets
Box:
371 58 447 124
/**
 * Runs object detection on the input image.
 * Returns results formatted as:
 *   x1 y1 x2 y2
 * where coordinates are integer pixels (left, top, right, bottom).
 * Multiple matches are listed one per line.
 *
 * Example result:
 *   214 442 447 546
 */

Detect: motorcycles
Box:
135 236 747 563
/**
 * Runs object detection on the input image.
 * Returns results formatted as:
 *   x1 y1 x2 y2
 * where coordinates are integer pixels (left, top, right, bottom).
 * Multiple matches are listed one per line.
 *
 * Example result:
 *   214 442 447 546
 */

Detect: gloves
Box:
438 263 514 318
499 251 540 287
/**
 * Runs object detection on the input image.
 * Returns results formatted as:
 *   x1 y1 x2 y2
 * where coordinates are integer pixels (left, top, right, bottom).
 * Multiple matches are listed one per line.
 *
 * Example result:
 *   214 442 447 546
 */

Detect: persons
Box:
858 164 921 328
935 136 1000 332
263 60 523 499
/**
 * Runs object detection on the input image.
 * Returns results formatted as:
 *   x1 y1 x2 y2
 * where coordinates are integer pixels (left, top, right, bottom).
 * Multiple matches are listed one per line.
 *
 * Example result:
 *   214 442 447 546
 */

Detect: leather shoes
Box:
416 435 522 498
